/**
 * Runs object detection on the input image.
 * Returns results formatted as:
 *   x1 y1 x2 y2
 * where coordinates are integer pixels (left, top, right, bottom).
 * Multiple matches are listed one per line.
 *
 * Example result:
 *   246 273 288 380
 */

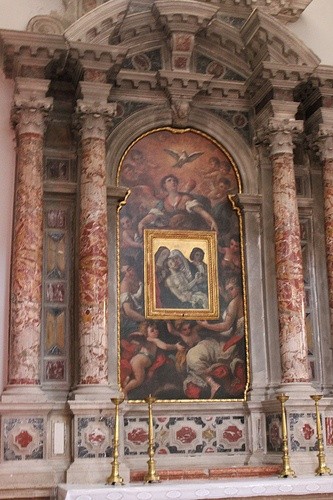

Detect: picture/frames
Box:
143 229 219 320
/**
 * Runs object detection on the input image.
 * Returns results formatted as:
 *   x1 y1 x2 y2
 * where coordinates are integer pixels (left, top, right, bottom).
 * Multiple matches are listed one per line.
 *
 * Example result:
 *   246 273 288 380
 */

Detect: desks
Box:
58 474 333 500
